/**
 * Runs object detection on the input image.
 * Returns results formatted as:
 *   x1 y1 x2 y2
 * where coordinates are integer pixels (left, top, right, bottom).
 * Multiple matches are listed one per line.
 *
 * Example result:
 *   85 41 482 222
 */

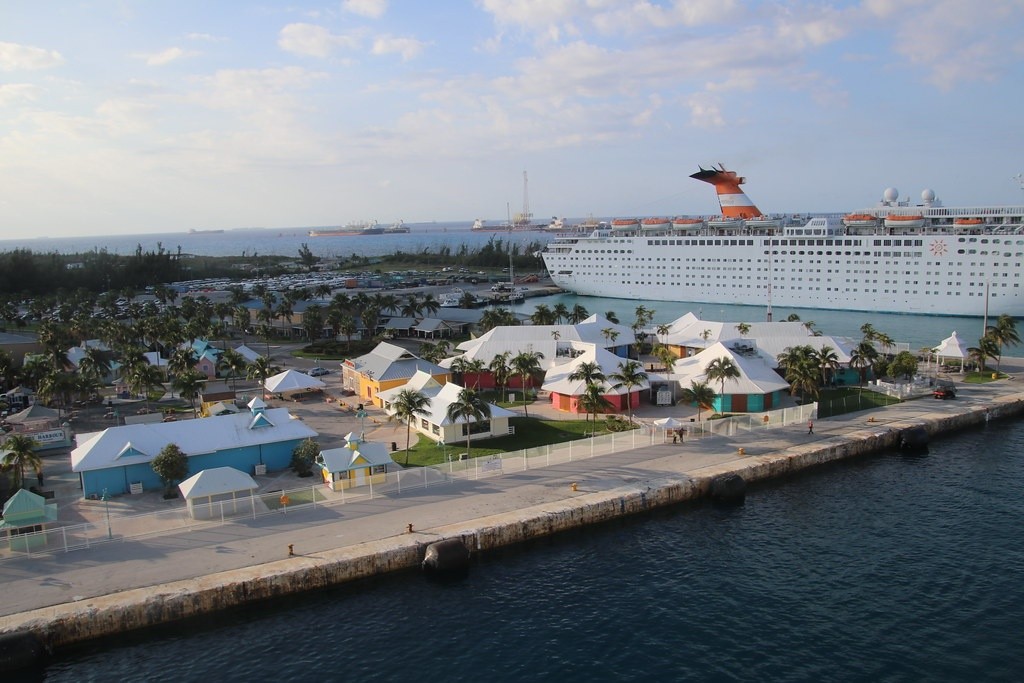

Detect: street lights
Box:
231 364 236 398
315 357 323 381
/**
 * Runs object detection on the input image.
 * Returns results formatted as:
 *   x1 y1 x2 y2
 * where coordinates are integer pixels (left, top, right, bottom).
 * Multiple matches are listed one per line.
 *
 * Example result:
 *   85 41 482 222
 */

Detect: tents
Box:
654 417 682 443
258 369 327 400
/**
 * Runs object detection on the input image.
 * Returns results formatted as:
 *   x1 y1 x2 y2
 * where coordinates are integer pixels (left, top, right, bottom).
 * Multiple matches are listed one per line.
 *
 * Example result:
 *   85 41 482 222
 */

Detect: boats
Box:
744 217 782 229
639 218 670 231
840 214 877 228
706 215 743 229
308 220 384 238
440 298 461 309
951 218 987 230
610 218 640 232
881 213 926 229
384 222 411 234
671 219 704 230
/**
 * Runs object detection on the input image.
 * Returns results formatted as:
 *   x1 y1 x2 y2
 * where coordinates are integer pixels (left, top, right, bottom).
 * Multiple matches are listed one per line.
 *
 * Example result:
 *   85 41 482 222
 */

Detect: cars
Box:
307 367 331 377
7 297 182 322
144 266 486 292
502 267 510 272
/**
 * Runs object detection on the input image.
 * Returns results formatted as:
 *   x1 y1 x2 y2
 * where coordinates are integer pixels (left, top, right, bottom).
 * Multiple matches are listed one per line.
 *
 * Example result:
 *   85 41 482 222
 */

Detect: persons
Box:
679 428 684 443
673 430 677 444
807 420 814 434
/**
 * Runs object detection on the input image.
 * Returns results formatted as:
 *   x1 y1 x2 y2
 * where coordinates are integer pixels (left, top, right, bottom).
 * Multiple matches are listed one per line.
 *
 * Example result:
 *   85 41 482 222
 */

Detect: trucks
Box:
491 281 516 292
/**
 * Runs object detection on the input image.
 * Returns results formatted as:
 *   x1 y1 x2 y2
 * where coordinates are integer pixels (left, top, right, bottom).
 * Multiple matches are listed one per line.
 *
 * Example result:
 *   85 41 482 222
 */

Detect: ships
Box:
540 162 1024 318
